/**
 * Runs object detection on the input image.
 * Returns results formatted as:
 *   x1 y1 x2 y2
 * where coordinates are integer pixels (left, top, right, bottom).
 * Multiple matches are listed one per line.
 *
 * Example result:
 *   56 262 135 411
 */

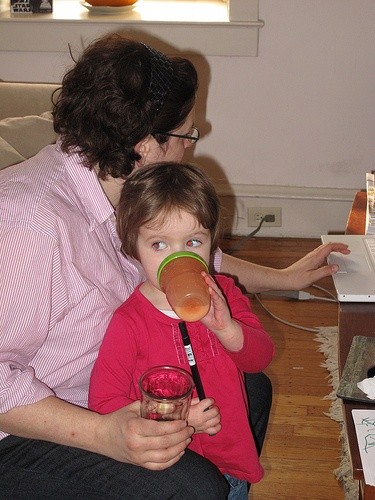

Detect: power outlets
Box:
247 207 282 227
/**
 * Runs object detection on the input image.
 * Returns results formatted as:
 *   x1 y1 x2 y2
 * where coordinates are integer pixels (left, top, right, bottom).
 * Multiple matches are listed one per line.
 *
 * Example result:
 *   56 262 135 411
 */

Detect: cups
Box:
137 365 195 429
157 252 211 322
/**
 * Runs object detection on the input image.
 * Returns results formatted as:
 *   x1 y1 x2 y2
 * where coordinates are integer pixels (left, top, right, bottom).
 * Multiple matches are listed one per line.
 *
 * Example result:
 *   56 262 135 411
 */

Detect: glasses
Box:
149 127 200 144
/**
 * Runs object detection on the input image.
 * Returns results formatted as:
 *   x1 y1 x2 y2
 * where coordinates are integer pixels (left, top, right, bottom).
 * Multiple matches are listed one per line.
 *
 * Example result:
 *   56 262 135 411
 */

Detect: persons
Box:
88 161 275 500
0 35 350 500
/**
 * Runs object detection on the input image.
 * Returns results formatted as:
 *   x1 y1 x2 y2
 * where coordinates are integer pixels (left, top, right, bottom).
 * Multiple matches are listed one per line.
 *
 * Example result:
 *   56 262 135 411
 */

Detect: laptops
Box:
321 235 375 302
